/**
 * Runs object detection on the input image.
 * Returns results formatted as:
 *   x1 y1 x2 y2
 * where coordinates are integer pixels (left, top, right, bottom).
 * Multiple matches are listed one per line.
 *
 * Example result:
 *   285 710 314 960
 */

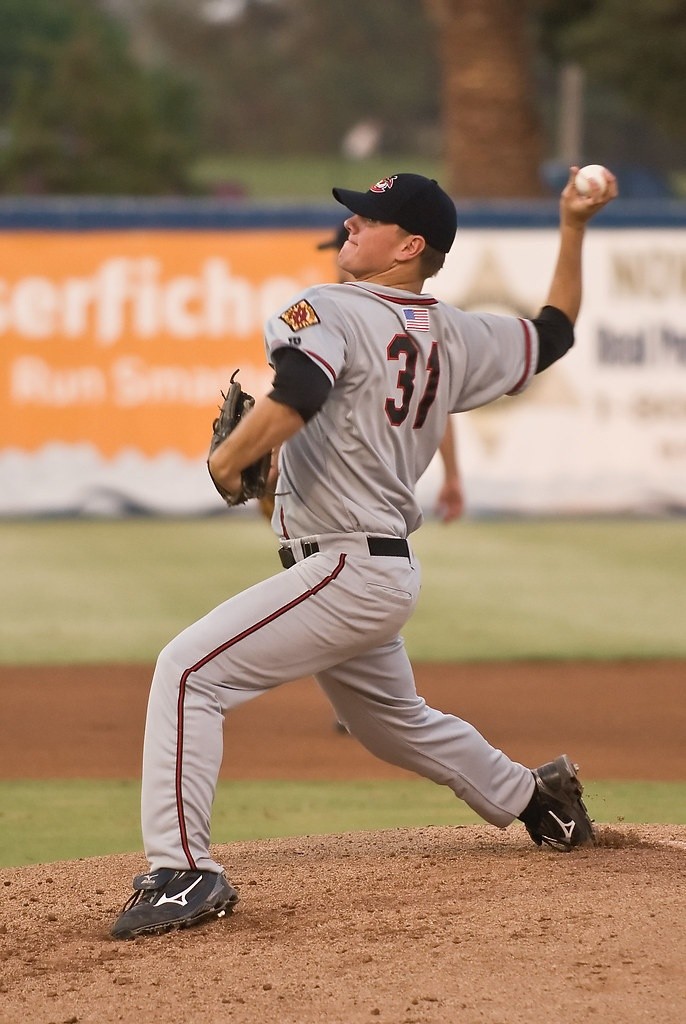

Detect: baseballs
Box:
576 164 610 197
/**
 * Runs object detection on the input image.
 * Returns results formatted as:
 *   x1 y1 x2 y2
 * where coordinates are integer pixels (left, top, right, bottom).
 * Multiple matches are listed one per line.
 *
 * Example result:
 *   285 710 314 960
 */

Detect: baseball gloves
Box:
210 366 272 506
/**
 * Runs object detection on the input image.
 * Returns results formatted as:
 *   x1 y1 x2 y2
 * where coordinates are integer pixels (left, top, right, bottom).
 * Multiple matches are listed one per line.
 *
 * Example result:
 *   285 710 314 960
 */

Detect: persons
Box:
107 166 617 940
259 225 465 735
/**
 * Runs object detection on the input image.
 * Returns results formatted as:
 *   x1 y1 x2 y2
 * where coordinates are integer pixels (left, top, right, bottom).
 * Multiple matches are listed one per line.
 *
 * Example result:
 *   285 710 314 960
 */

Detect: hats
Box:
333 173 458 253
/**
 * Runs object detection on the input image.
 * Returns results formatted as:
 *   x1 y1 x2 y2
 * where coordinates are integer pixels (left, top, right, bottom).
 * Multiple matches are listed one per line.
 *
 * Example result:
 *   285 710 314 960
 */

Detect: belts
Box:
278 538 410 568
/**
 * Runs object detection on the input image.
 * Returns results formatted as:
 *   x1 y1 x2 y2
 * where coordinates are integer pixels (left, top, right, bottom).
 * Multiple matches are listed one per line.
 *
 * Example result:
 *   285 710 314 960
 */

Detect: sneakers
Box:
110 868 241 940
527 754 597 854
